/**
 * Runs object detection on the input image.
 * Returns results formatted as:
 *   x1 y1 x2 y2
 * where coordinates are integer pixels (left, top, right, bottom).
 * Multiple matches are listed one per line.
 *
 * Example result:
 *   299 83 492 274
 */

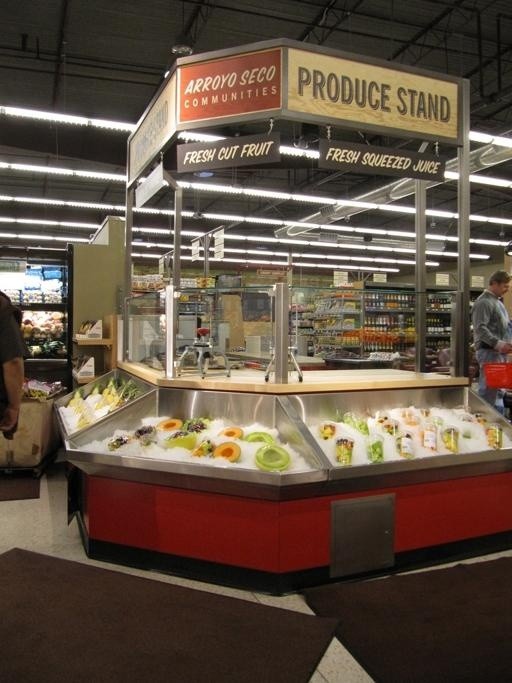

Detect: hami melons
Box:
158 418 182 433
212 441 240 462
219 427 243 437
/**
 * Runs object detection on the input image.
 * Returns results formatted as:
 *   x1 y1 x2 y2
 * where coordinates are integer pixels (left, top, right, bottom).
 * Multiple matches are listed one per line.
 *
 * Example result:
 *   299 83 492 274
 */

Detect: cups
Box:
319 399 502 465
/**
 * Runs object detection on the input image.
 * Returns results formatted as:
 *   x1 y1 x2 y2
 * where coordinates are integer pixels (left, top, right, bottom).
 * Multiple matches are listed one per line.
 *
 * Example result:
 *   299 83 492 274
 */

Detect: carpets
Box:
2 546 342 681
304 555 511 682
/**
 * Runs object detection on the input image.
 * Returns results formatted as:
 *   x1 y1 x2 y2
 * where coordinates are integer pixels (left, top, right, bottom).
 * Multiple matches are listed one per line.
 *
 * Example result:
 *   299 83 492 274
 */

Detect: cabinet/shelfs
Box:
73 314 117 389
0 242 71 392
288 282 486 365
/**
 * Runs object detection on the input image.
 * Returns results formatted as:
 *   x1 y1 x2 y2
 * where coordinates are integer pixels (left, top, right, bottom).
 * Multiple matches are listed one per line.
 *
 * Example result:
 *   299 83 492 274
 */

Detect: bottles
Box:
364 313 444 333
306 340 314 356
366 293 450 308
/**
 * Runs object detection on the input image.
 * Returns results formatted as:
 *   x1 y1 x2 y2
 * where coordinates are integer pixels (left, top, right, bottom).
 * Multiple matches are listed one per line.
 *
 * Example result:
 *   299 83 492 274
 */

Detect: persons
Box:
472 269 511 417
1 290 35 439
